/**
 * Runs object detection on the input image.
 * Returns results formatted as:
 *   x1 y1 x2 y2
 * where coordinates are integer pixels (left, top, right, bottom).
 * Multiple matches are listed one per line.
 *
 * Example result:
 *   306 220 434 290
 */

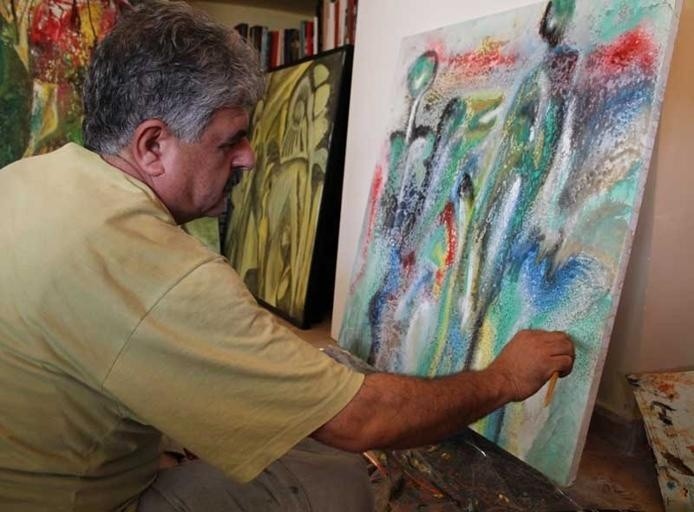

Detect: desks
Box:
310 341 667 511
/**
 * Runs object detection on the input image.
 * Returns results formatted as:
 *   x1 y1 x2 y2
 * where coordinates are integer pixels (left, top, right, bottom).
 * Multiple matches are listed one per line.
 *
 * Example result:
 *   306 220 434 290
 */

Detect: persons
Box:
1 1 576 511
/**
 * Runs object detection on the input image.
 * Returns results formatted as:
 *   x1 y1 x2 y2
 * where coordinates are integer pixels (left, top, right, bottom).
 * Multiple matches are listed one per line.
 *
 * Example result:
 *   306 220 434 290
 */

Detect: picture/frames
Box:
221 46 353 330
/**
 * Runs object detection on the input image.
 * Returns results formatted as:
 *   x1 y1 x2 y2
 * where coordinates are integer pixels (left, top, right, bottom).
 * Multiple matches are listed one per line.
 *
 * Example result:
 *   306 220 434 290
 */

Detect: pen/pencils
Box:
543 371 562 407
